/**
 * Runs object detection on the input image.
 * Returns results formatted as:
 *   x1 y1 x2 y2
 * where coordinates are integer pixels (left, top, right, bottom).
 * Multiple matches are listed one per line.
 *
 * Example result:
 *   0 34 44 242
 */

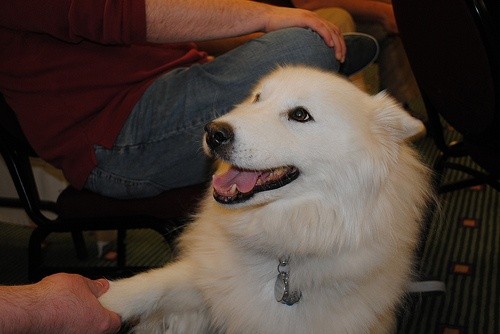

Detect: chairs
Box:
0 93 200 271
391 0 500 312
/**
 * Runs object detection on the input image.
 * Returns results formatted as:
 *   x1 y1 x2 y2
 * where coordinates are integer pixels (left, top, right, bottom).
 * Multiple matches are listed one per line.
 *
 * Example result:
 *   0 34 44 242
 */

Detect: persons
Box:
252 0 428 122
0 0 446 296
0 273 122 334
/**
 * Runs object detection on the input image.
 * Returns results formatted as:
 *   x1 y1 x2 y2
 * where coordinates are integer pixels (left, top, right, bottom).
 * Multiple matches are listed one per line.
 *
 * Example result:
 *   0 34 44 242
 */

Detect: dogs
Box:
97 62 436 334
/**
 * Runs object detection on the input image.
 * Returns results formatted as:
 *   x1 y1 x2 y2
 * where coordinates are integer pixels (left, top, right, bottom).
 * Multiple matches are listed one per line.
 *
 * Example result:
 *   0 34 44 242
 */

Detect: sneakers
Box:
335 32 380 77
